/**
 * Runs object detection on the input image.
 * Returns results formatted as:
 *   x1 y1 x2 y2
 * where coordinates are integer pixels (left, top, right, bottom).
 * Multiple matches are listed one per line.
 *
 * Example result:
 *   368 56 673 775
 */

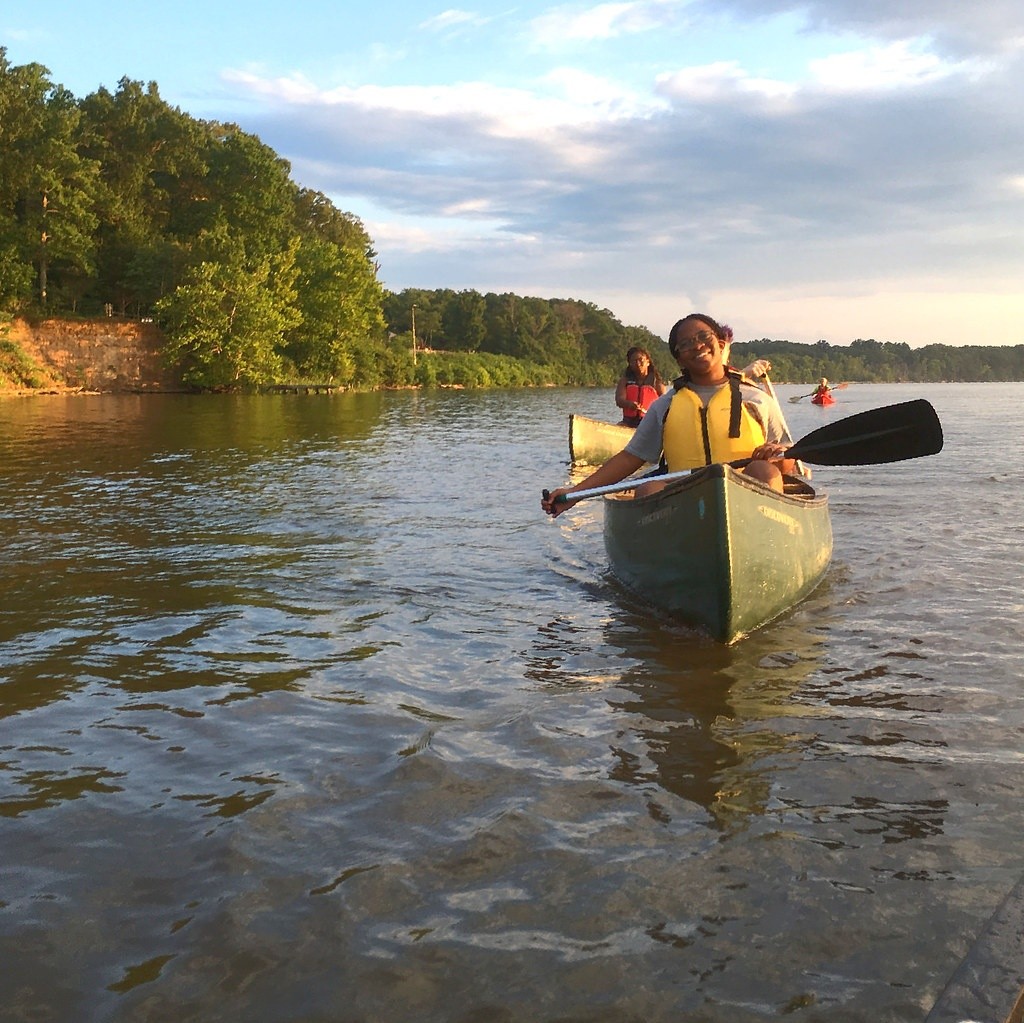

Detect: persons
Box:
811 378 831 399
541 313 795 518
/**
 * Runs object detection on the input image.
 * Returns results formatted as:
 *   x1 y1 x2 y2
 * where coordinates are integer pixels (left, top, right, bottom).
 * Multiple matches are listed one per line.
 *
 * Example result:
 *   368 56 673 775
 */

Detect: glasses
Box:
674 330 719 352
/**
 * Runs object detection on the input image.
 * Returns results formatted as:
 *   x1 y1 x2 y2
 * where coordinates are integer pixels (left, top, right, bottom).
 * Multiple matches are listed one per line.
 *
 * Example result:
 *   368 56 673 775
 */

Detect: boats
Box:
811 394 834 407
601 464 833 644
569 414 637 468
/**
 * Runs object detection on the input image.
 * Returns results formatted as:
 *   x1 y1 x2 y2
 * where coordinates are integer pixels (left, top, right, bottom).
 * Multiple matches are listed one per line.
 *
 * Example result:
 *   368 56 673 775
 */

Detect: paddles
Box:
791 383 847 402
541 397 944 511
761 371 804 476
